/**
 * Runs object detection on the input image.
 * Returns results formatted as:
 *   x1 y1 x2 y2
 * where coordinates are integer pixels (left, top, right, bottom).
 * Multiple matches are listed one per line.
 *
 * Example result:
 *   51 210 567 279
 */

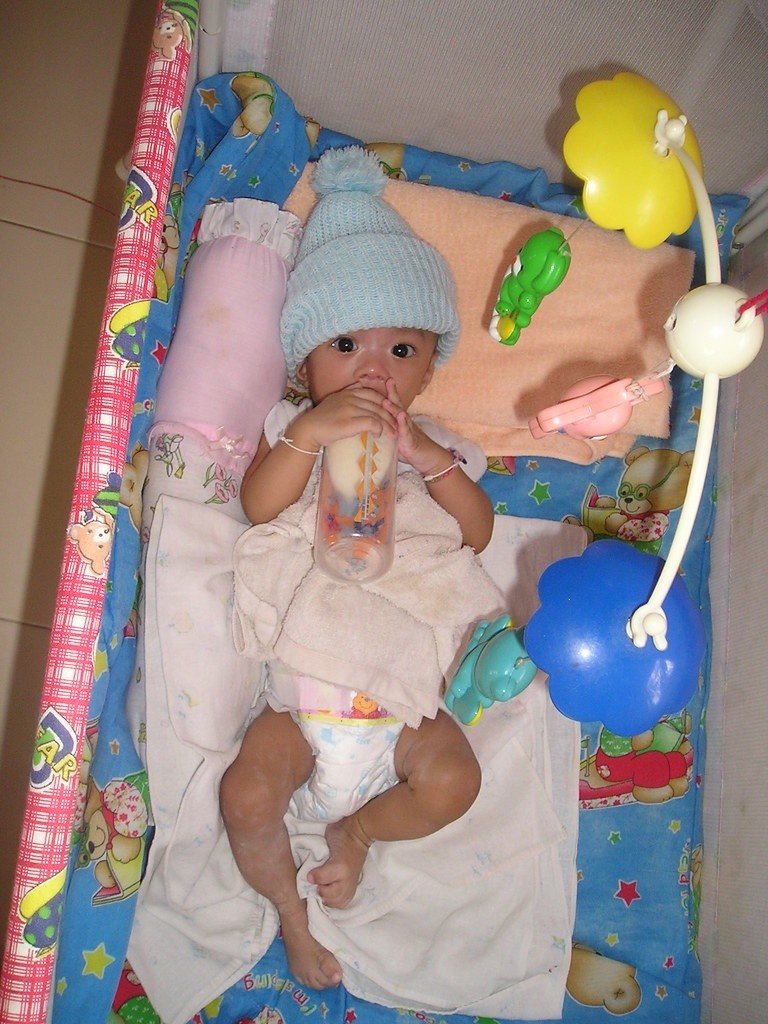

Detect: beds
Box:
0 0 768 1024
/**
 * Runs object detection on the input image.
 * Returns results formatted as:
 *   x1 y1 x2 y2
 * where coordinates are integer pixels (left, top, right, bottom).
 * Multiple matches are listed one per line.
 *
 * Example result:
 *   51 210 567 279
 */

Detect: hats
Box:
279 144 460 389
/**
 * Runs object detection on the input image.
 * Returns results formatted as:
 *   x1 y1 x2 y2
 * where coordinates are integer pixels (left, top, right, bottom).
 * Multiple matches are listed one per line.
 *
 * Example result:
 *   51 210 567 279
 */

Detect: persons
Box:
218 224 497 990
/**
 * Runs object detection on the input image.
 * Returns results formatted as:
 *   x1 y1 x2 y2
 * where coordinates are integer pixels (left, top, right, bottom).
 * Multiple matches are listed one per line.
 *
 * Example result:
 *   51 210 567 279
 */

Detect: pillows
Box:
151 197 306 478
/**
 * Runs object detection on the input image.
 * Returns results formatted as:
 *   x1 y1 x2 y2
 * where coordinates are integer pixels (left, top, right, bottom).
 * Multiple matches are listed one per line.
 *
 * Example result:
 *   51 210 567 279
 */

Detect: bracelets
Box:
277 434 324 457
421 447 467 484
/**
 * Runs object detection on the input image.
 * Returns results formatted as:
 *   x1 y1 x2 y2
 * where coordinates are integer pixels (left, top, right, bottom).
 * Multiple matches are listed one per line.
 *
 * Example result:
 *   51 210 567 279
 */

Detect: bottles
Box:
313 419 398 585
